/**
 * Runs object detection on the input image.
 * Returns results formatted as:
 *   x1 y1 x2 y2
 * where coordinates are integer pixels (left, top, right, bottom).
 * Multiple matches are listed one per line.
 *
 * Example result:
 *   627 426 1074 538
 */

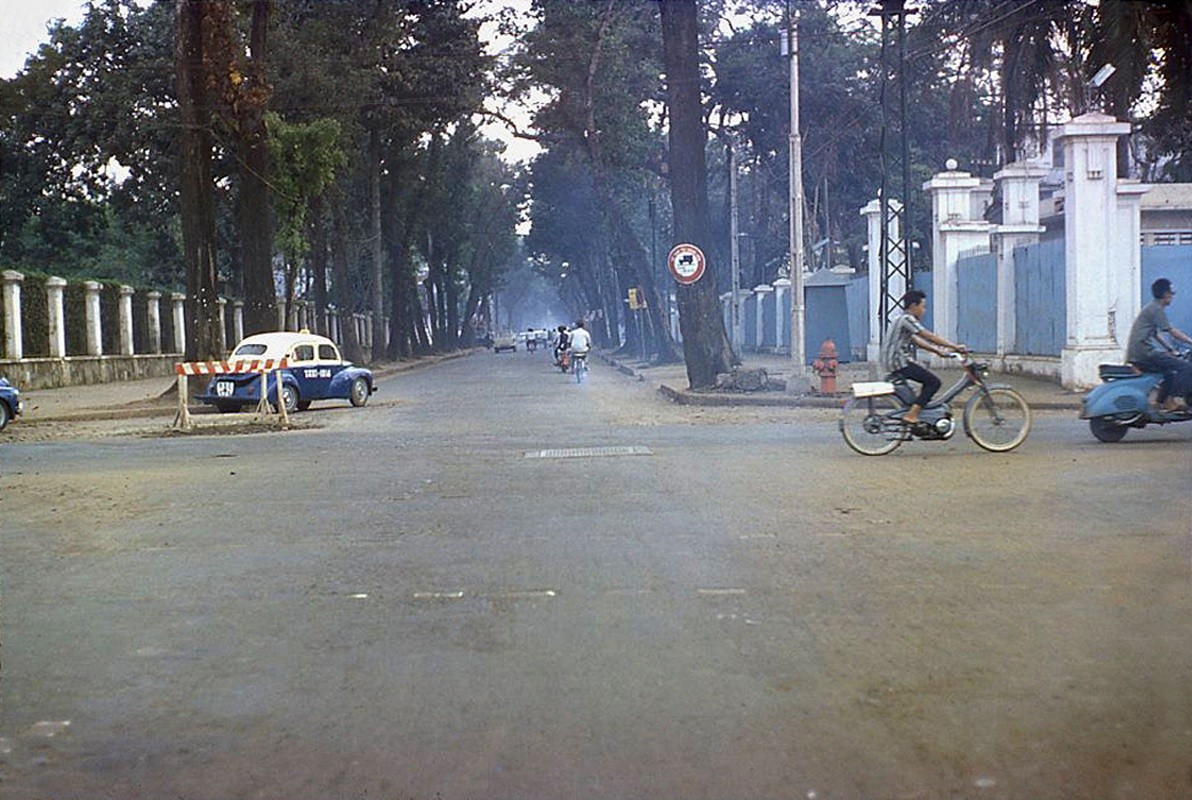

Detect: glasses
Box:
1166 290 1176 296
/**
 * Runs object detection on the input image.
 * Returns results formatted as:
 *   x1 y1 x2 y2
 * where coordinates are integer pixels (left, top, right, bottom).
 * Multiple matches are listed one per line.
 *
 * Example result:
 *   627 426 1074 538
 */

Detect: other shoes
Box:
569 369 573 374
585 365 590 370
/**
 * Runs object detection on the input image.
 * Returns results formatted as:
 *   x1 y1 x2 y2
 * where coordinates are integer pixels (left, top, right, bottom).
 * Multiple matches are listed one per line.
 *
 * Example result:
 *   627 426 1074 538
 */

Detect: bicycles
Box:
574 352 587 382
835 347 1035 455
529 341 535 353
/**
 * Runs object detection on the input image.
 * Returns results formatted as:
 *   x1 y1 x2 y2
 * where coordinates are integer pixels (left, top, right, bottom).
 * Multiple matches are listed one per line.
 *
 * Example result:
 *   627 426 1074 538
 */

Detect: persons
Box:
542 328 548 348
526 328 537 351
1126 278 1192 411
550 325 570 366
567 319 592 370
883 291 967 424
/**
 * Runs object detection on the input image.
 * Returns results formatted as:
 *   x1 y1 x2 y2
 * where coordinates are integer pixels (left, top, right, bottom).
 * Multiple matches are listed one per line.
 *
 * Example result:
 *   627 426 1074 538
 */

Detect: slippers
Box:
1161 404 1192 414
901 418 932 428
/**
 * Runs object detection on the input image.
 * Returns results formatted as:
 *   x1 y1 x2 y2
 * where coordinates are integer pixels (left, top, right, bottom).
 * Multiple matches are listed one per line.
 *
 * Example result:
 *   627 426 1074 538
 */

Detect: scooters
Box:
558 352 572 373
1077 349 1192 444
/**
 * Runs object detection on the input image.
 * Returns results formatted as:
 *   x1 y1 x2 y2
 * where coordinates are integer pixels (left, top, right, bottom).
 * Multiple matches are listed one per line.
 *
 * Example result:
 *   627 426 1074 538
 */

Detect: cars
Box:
492 330 517 353
0 372 24 430
191 329 379 414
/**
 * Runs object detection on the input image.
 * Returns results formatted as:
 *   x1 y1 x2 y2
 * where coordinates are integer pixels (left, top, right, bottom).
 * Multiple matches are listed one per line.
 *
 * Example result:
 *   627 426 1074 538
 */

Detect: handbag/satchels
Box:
562 354 571 369
